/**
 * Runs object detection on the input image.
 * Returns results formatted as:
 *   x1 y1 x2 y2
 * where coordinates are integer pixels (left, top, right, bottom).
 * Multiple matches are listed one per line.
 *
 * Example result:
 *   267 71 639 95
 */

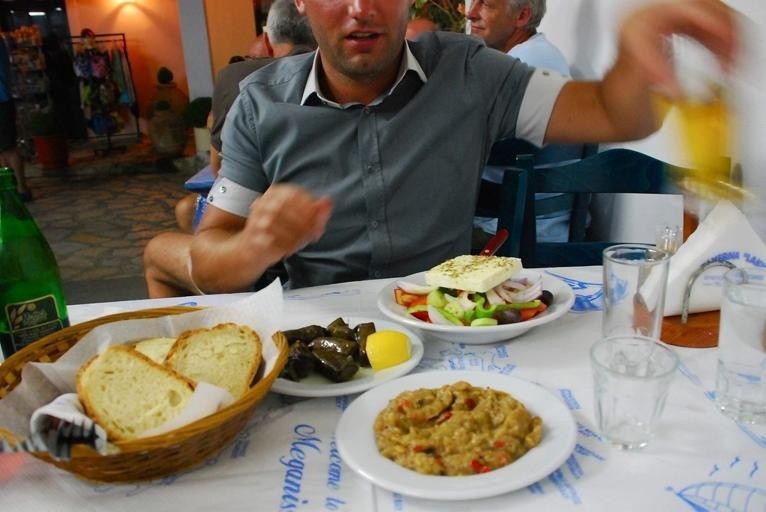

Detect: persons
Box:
243 33 270 60
465 0 577 265
189 0 737 296
142 0 319 298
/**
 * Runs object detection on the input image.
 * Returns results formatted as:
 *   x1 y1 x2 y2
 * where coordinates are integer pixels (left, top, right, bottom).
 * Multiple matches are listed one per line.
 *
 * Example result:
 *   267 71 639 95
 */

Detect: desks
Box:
184 159 223 235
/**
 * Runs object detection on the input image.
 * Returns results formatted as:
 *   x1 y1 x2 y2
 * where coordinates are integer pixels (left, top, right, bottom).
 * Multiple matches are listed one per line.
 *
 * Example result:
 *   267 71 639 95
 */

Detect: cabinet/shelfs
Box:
1 0 86 161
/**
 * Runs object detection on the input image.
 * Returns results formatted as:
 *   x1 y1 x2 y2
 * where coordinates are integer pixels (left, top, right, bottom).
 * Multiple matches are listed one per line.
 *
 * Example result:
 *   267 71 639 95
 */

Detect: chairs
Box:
471 137 742 269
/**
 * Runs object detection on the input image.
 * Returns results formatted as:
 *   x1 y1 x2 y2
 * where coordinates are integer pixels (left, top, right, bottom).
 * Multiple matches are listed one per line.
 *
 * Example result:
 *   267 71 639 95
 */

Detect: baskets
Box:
0 305 288 484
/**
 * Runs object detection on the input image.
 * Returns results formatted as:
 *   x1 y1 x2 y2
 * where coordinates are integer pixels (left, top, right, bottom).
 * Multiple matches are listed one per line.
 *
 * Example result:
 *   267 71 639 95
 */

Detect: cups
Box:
603 244 670 342
590 336 675 451
718 267 765 361
712 360 766 424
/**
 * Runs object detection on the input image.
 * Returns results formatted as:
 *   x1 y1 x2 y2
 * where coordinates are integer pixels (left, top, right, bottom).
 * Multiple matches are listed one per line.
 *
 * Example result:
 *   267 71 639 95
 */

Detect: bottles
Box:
0 166 71 358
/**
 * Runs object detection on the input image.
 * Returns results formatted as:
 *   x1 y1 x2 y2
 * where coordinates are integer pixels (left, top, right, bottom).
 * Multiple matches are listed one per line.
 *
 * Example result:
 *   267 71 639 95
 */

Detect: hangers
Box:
74 38 118 54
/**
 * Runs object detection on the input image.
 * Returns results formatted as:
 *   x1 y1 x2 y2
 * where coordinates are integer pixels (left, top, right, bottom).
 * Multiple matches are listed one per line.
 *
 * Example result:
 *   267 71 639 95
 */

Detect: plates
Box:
269 317 424 397
335 370 577 501
377 271 575 347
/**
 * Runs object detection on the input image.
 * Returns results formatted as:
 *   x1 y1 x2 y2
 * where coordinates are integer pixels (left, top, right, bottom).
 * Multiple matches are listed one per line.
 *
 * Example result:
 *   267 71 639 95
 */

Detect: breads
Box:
425 253 523 293
76 321 262 442
